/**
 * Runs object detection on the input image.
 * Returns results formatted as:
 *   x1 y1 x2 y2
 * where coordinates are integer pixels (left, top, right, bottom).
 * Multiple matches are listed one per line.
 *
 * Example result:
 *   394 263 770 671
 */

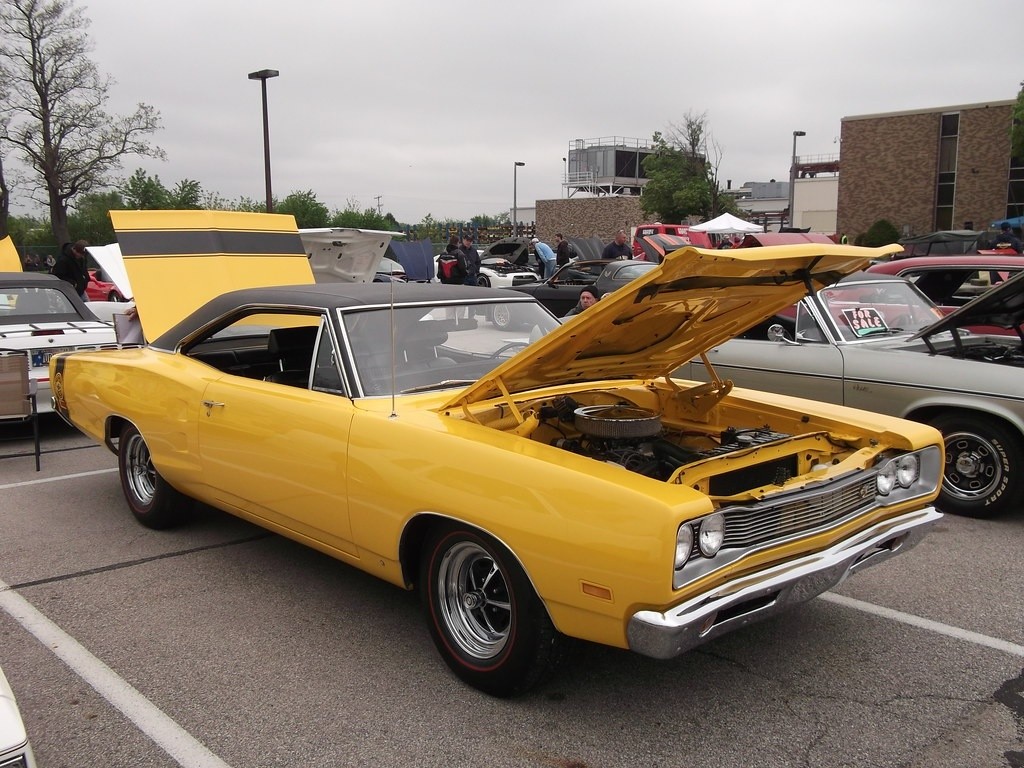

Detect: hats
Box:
581 286 599 298
465 235 474 242
531 238 539 243
1001 223 1010 231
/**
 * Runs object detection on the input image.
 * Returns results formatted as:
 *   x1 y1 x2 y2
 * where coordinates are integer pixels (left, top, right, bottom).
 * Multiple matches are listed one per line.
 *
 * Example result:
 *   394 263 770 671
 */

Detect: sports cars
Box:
46 208 948 703
501 270 1024 522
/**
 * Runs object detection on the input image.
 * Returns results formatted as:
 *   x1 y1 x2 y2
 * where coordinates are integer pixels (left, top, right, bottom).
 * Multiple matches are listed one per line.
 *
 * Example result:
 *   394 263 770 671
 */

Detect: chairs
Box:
393 320 459 386
267 325 341 392
0 348 41 472
9 291 49 315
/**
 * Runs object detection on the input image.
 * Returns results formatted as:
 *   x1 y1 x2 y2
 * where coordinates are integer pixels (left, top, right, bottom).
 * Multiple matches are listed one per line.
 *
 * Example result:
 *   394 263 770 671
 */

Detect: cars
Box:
735 232 1024 338
79 269 126 302
0 269 117 417
248 222 717 333
78 241 135 324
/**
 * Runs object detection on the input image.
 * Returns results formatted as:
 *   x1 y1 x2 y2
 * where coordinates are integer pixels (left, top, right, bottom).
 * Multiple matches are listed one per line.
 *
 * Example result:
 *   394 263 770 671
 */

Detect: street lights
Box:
512 161 525 237
786 129 807 227
247 68 280 213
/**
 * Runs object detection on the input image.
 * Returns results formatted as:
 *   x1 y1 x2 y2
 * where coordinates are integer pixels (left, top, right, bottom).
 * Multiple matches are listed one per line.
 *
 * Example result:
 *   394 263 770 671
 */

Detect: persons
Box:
529 234 570 279
602 229 632 272
987 221 1023 285
438 234 481 286
23 240 91 302
564 286 612 317
